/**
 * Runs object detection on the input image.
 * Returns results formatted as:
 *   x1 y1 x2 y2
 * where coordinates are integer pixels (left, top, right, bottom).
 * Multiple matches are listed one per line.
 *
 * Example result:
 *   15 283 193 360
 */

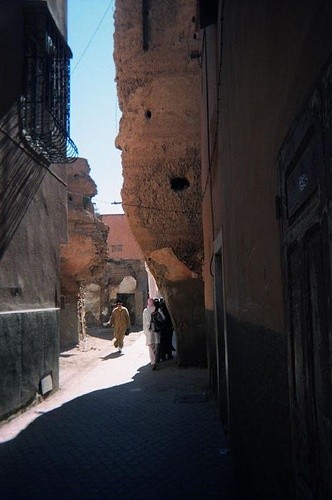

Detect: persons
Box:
142 297 176 371
107 298 130 353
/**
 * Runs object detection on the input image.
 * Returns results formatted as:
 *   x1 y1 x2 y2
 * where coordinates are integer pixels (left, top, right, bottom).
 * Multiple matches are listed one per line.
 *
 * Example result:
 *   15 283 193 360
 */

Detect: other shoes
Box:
152 364 160 371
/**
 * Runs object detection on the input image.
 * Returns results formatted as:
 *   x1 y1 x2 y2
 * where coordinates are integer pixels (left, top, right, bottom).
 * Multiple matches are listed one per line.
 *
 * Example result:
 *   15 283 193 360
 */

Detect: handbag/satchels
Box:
149 307 165 333
